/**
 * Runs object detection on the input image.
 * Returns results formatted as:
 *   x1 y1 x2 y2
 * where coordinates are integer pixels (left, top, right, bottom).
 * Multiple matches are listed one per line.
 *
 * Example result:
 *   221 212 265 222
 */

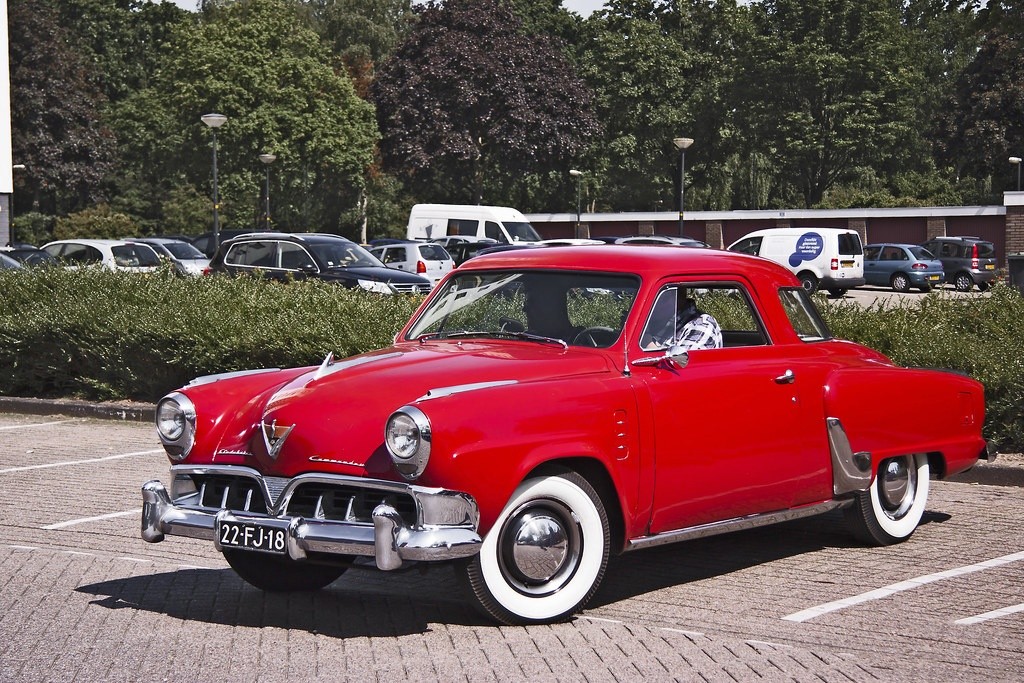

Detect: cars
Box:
338 235 713 295
140 243 998 627
21 238 174 279
904 235 998 293
192 228 276 259
0 242 58 280
862 243 945 293
120 236 211 280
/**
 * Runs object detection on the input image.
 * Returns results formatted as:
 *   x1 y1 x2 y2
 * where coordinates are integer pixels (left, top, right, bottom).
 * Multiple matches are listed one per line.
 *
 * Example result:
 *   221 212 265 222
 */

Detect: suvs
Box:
201 233 432 297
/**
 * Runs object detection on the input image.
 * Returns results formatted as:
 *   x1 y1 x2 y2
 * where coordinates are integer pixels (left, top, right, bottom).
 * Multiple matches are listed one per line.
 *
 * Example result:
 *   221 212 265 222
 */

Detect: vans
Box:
726 228 866 298
402 200 547 268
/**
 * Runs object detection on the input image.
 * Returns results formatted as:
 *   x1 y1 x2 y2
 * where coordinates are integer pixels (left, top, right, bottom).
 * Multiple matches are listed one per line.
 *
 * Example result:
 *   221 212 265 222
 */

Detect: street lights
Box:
1009 156 1022 191
257 153 276 233
673 137 695 237
200 113 227 254
9 164 28 246
568 169 582 239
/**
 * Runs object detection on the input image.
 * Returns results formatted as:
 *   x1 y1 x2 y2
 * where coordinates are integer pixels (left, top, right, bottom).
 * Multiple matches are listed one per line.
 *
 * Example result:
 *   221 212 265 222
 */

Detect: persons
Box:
620 286 724 351
